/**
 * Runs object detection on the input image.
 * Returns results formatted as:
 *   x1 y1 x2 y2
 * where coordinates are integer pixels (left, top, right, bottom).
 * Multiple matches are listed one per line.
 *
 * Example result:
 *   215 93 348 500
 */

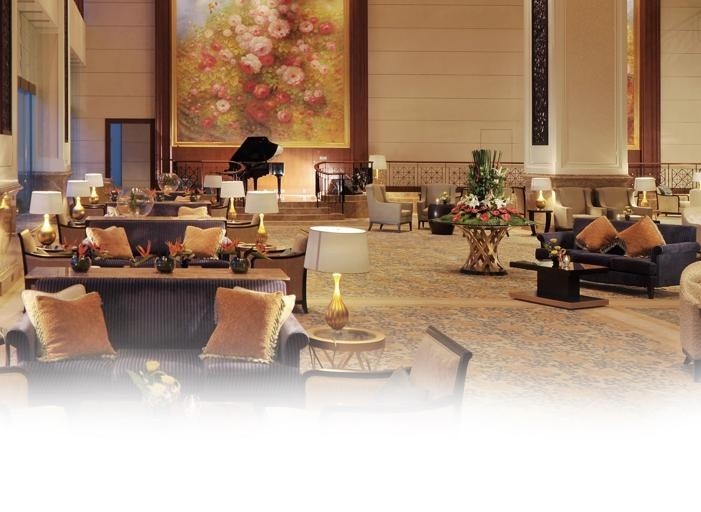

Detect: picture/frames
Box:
170 0 351 149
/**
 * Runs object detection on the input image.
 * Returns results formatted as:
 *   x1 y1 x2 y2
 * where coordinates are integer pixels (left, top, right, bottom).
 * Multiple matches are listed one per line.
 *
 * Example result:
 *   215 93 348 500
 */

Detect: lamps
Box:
368 155 388 179
85 174 105 203
204 175 222 206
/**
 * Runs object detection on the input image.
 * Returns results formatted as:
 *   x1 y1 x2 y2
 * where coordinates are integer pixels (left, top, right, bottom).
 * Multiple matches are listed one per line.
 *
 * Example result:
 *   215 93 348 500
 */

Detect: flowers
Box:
429 149 537 226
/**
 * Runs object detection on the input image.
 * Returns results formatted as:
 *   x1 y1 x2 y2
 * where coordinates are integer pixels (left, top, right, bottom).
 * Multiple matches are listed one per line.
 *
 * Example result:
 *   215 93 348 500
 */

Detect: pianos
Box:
225 136 286 197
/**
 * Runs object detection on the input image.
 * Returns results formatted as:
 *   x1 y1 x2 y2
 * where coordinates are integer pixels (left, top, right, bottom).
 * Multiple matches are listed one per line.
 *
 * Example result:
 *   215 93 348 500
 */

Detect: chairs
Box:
0 178 701 413
